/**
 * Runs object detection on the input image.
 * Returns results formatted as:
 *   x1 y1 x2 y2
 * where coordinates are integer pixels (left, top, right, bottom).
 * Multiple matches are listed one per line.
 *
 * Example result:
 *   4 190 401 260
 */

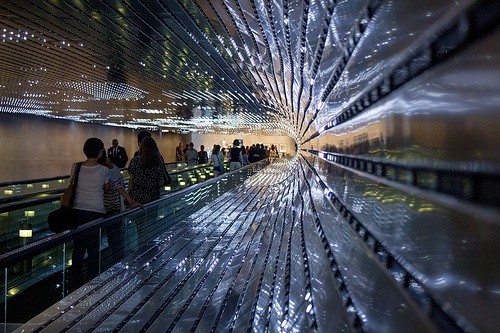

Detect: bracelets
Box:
129 200 135 204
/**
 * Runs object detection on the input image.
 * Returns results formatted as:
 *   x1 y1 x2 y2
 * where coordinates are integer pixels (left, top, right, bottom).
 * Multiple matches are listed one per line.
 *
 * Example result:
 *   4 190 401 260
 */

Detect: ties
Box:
114 147 116 157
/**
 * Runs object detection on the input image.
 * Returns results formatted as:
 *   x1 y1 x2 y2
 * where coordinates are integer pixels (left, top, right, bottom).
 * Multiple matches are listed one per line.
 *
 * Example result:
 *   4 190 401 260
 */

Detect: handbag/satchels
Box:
48 209 73 232
220 165 224 173
60 163 78 206
227 159 230 167
160 156 171 186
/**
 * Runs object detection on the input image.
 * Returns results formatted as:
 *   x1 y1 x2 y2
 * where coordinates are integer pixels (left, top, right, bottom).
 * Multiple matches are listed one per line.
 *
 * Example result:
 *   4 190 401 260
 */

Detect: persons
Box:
70 130 170 291
176 139 278 177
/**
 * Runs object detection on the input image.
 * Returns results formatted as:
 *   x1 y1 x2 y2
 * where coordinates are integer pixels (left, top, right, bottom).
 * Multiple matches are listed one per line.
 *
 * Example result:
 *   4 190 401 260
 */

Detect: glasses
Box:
102 148 105 151
